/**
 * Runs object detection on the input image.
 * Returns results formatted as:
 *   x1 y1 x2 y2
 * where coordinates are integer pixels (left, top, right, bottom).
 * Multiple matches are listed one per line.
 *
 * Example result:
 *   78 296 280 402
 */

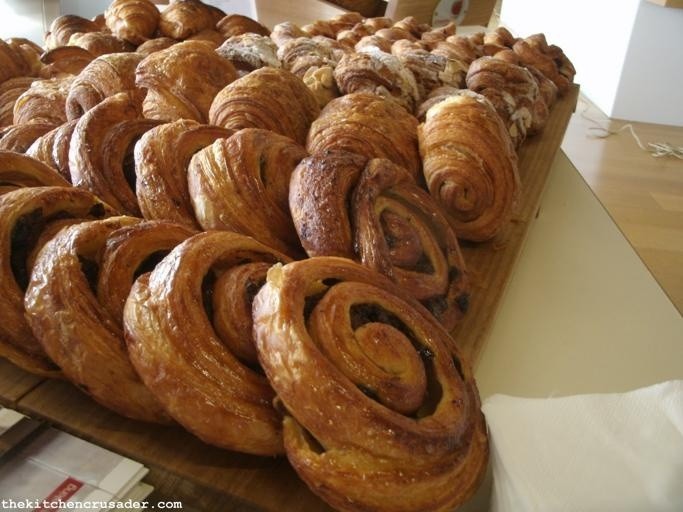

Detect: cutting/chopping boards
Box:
1 82 581 511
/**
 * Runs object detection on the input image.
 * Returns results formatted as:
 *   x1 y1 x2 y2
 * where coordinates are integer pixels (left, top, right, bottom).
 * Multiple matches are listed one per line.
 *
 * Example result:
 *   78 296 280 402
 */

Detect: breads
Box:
135 41 236 125
528 35 582 80
306 91 427 183
270 20 303 44
64 93 138 213
2 119 55 158
68 28 117 59
211 8 266 35
2 37 140 124
250 255 489 512
27 118 76 183
334 49 417 120
206 66 314 141
277 35 335 75
188 127 311 259
120 229 294 459
38 17 96 49
461 58 546 134
333 12 515 93
214 31 277 76
298 18 353 33
132 118 214 234
157 1 225 40
514 38 571 97
22 219 179 432
418 93 522 244
106 0 160 40
294 62 332 92
0 151 71 202
132 39 174 62
287 150 469 338
495 49 557 115
0 187 119 378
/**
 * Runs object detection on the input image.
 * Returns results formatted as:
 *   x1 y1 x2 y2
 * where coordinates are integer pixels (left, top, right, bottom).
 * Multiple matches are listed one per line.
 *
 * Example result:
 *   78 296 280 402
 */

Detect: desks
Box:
0 147 681 511
